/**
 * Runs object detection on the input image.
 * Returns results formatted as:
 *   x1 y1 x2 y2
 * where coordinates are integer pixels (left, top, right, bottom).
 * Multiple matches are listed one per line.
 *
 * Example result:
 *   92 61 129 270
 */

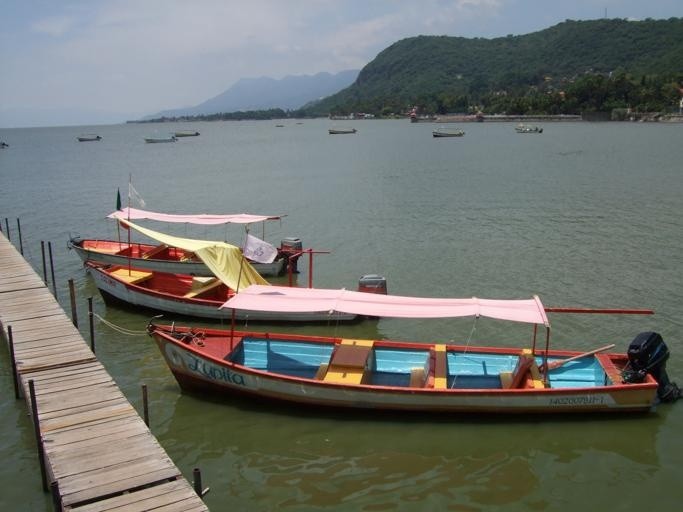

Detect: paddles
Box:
539 344 615 373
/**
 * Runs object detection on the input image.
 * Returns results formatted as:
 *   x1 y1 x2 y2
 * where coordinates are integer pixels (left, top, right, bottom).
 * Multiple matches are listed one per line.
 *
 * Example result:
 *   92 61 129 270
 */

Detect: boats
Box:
146 283 679 421
83 175 386 324
144 131 199 144
432 127 465 137
329 127 357 134
77 134 102 142
515 124 543 134
68 206 305 277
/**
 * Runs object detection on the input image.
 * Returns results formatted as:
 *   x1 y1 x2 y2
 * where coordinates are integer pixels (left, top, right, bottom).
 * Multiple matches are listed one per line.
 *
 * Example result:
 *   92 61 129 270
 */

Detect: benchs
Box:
409 344 447 388
180 252 195 261
183 280 221 298
143 245 169 259
500 348 544 389
